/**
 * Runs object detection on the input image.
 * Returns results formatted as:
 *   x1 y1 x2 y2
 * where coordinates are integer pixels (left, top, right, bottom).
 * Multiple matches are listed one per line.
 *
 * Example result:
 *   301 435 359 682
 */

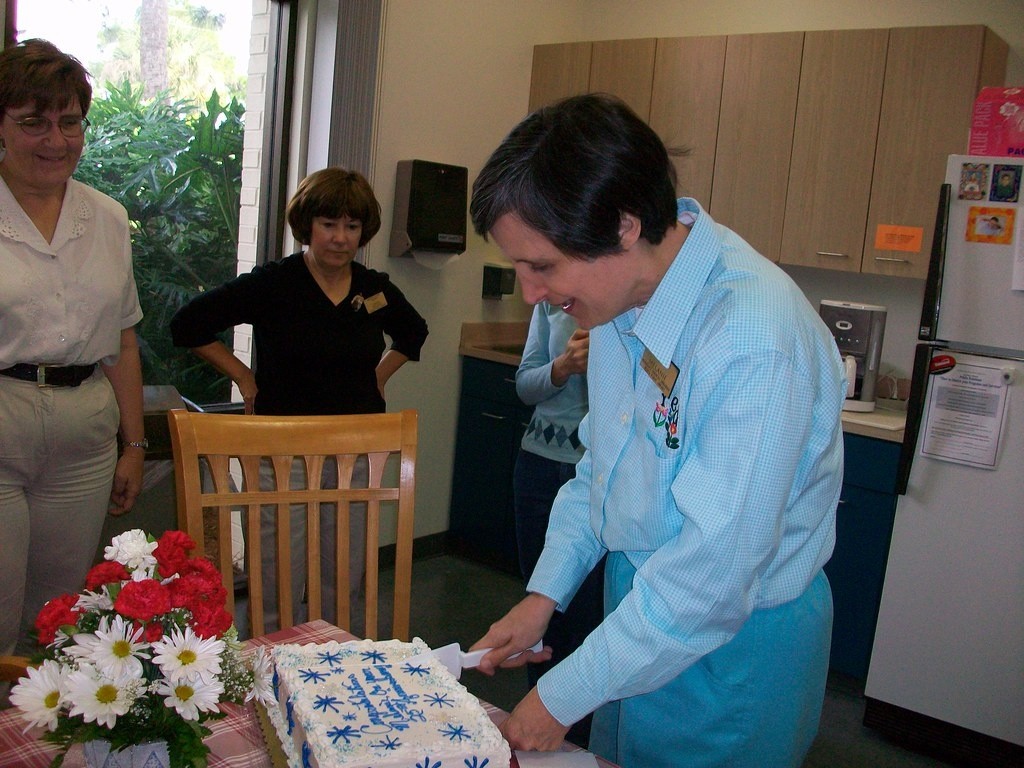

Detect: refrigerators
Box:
861 151 1024 768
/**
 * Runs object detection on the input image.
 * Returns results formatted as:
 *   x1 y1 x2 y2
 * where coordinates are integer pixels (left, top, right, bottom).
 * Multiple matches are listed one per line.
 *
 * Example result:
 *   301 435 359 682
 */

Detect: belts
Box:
0 363 96 387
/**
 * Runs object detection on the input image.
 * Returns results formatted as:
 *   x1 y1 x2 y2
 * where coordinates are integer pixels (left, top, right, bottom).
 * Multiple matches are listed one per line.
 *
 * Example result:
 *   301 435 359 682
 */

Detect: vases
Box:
81 738 171 768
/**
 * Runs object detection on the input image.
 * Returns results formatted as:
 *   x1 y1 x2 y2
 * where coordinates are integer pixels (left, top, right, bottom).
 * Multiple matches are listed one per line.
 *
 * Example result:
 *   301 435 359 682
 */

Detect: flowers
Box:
9 529 278 768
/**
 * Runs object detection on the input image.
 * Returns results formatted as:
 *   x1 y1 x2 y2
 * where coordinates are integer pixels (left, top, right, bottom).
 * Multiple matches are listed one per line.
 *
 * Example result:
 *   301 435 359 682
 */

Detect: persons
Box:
967 171 1013 236
467 93 844 768
513 298 603 749
170 168 429 638
0 38 149 708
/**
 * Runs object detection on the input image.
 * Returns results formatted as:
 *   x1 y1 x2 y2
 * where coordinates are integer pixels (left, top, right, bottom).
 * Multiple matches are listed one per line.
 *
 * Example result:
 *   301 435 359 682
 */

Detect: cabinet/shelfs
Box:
445 356 530 580
822 433 901 682
526 24 1010 278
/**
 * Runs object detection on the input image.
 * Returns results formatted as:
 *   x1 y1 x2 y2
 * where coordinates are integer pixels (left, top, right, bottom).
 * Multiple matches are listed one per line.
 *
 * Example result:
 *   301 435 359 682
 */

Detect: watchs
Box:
122 438 149 450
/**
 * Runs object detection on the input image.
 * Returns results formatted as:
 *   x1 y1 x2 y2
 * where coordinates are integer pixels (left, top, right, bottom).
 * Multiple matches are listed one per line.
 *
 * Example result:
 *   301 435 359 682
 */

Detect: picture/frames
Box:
990 164 1022 203
965 206 1015 244
958 162 991 201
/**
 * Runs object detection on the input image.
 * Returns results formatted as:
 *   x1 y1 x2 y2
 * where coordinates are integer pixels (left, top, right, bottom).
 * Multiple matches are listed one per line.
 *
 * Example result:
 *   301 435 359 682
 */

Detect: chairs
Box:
167 407 419 643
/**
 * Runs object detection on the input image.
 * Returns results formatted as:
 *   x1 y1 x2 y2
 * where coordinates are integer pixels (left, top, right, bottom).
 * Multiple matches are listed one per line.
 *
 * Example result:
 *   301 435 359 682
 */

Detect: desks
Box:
0 618 627 768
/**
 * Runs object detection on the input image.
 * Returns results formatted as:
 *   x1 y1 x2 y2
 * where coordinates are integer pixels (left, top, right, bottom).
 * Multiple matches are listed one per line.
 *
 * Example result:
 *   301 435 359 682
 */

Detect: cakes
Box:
264 637 512 768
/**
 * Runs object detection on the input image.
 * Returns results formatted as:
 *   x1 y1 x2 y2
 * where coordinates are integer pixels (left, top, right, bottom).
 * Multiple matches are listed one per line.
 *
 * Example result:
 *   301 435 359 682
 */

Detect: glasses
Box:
4 112 91 137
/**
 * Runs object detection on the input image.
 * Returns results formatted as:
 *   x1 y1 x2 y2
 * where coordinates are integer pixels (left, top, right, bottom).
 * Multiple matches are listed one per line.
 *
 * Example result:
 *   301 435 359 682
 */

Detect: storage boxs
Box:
969 86 1024 158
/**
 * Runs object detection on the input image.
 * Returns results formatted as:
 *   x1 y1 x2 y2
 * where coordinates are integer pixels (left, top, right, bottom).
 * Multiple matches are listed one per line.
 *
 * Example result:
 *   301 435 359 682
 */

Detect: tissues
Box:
386 159 469 272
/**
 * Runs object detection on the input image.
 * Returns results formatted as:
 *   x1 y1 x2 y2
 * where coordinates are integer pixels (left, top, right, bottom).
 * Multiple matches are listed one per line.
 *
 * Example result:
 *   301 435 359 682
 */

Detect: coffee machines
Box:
819 298 888 412
